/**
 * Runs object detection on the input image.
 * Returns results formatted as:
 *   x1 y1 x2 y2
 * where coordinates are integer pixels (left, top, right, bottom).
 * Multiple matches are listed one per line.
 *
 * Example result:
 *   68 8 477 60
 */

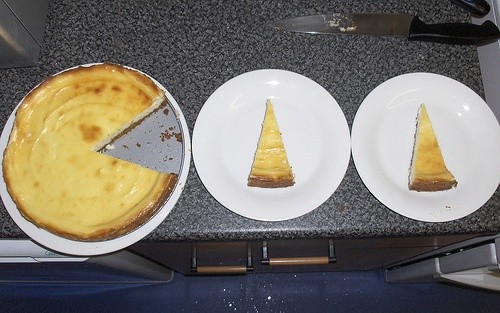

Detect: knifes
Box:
266 12 500 45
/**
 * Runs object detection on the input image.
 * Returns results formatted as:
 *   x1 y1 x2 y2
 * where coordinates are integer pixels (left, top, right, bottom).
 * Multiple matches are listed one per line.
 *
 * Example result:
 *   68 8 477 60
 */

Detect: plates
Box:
0 62 191 257
351 72 500 222
191 68 351 222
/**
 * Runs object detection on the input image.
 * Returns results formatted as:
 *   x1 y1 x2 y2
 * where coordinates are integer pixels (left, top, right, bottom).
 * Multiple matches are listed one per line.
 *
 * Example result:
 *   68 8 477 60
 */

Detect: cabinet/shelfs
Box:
0 0 500 294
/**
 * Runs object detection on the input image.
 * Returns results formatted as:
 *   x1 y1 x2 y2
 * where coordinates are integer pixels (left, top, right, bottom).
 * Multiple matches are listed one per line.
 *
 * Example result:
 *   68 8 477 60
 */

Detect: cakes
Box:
408 104 458 192
1 61 178 245
247 98 294 187
